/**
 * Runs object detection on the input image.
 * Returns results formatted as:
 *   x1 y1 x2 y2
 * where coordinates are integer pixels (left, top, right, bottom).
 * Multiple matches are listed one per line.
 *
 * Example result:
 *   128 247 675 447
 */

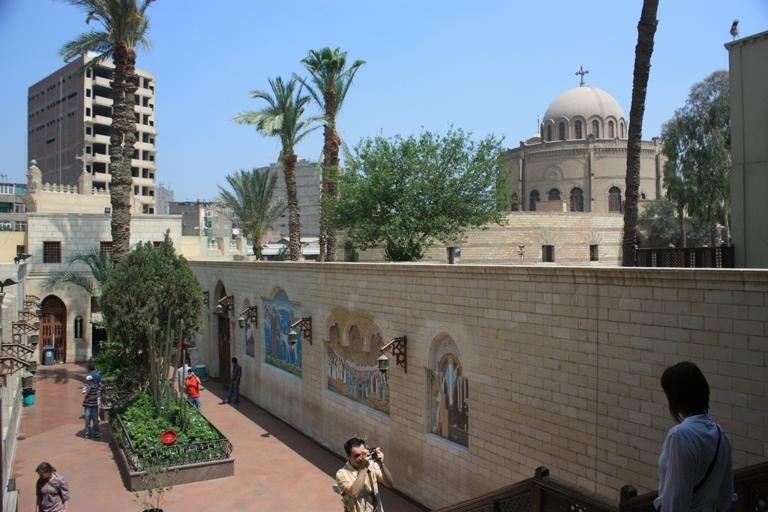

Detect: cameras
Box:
366 447 377 460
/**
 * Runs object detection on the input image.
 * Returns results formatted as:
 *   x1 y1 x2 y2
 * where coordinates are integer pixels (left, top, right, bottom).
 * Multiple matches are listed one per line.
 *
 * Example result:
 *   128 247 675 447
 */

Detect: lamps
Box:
286 316 313 350
376 336 407 375
213 295 258 330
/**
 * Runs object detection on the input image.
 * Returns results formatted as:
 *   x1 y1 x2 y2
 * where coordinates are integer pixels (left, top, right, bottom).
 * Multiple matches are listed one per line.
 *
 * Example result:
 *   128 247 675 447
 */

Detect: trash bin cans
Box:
22 388 35 405
196 365 206 377
43 344 55 365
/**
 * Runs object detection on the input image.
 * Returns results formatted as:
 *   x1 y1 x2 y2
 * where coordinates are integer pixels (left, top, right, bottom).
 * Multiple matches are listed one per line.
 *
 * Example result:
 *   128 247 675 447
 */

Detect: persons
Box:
338 438 394 511
175 357 206 411
34 462 72 512
81 363 103 439
651 360 736 512
225 353 246 408
